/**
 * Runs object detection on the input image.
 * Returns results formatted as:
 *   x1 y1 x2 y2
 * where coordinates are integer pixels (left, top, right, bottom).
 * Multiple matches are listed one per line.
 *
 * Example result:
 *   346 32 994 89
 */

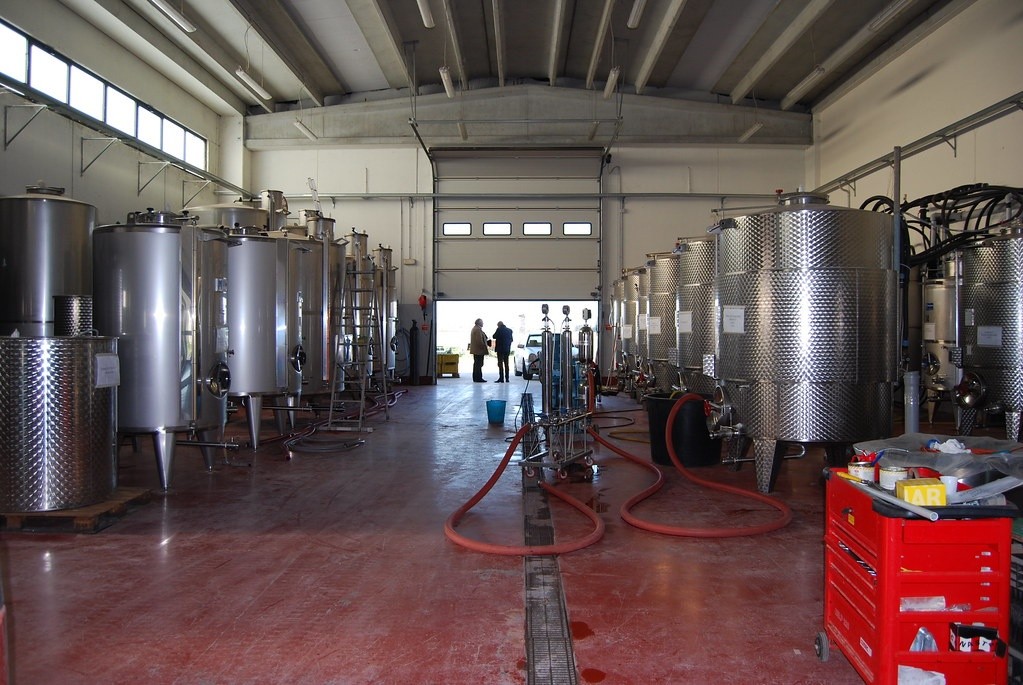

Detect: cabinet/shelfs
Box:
813 467 1021 685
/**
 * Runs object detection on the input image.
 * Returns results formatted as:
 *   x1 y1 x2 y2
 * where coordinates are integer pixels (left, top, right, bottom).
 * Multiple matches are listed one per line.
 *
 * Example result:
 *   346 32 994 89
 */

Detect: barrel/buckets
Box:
486 400 507 424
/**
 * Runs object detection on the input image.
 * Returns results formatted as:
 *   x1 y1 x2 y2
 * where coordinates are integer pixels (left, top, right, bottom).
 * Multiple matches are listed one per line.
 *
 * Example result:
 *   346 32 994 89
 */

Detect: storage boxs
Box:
895 480 946 506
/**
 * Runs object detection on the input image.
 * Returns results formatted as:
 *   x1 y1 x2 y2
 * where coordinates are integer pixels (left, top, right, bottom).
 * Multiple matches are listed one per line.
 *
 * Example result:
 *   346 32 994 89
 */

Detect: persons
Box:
493 321 513 383
470 319 489 382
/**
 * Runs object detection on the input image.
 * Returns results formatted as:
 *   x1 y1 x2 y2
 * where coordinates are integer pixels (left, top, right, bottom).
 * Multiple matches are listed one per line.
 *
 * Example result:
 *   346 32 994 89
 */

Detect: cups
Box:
940 476 958 494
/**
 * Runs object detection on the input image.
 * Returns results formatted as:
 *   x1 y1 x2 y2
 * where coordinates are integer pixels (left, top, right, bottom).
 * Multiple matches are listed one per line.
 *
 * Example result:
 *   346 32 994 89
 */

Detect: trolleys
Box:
518 411 594 482
547 362 600 439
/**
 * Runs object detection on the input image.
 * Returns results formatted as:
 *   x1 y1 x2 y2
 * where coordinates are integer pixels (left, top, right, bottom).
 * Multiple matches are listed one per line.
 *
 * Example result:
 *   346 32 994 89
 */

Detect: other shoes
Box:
506 379 509 382
473 378 487 382
494 378 504 383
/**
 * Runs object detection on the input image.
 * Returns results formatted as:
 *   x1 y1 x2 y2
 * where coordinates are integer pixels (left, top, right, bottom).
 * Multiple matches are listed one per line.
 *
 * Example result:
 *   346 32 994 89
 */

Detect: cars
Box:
513 332 579 380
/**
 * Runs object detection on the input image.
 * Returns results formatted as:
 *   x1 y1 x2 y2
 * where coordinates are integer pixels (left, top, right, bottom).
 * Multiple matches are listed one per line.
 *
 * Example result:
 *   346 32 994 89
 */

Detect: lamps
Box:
234 22 271 102
736 89 764 147
455 91 476 141
787 22 828 105
587 21 625 143
416 1 435 31
292 83 320 142
149 0 200 32
625 0 646 28
868 1 913 34
437 18 460 100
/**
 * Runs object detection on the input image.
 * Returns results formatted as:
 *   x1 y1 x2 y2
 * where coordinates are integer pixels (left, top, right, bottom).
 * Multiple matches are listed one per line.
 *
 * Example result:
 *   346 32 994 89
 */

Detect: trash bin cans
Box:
486 399 507 423
